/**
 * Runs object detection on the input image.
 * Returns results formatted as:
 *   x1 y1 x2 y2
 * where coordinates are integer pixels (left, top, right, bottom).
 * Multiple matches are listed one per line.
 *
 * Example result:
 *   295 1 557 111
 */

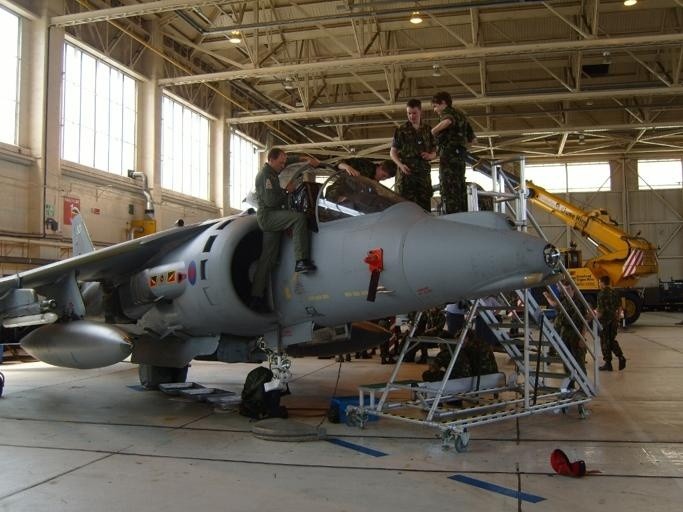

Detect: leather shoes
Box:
416 358 426 364
295 260 315 273
248 297 270 312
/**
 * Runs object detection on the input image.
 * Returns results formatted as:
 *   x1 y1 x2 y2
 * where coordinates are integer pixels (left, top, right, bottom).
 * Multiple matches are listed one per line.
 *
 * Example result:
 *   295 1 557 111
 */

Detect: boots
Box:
382 357 387 363
599 360 612 371
619 355 626 370
388 356 397 363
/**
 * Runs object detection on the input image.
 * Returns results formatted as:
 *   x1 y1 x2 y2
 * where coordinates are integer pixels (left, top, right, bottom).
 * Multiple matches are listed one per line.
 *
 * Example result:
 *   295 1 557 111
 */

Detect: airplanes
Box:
0 161 560 418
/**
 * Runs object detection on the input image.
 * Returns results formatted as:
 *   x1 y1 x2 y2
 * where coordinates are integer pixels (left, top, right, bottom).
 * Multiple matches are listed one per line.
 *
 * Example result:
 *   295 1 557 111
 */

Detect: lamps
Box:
432 63 442 77
623 0 637 6
285 81 294 90
586 99 595 107
598 51 613 65
229 30 241 45
578 135 585 145
349 147 357 155
409 10 423 24
324 117 331 123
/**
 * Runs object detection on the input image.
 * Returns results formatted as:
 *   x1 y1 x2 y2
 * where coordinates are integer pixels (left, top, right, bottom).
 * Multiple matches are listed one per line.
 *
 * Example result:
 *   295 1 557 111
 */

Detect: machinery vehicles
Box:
464 152 660 329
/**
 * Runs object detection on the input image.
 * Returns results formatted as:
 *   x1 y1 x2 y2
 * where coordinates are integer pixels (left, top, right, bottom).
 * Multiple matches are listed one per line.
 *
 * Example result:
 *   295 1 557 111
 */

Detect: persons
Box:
459 330 498 373
554 283 589 388
423 306 446 363
430 92 477 213
594 276 626 372
375 315 412 365
326 157 398 206
544 280 566 307
332 350 372 364
241 147 321 312
390 98 438 211
422 335 457 382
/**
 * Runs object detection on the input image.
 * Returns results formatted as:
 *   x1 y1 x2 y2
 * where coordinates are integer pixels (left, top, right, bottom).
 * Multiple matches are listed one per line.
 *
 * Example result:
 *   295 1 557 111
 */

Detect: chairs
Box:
303 179 326 220
